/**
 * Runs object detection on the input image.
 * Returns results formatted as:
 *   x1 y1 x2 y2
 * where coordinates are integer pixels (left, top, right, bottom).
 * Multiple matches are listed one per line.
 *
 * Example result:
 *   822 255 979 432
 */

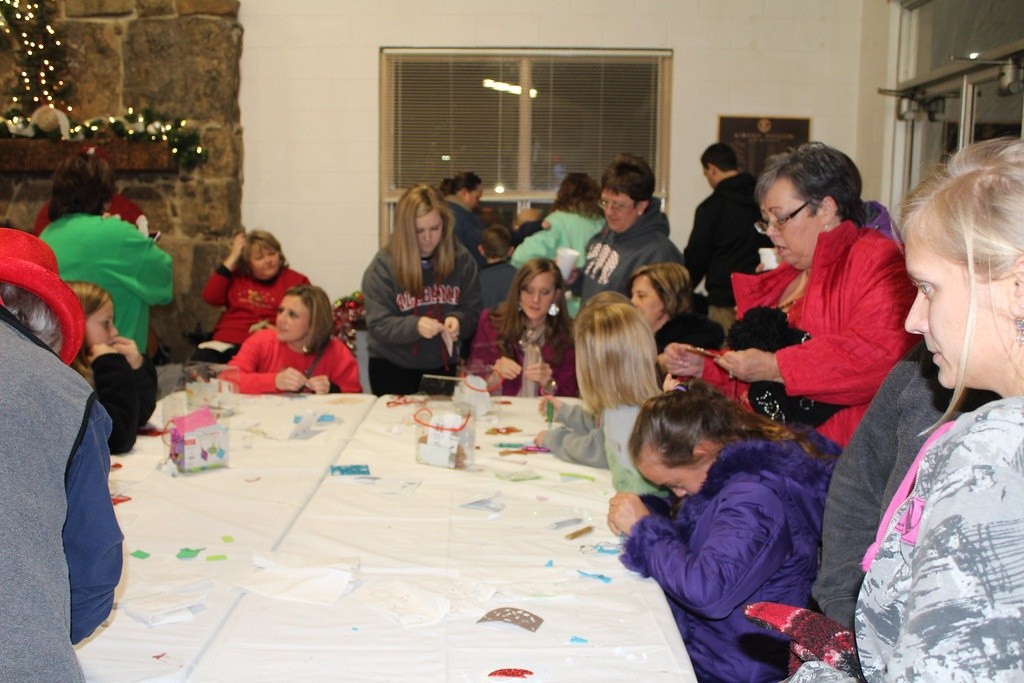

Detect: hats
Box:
727 305 853 428
0 227 86 365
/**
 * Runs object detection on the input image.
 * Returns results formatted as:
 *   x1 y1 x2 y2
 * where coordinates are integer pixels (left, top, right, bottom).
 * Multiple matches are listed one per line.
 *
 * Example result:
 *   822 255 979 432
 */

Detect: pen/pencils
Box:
547 396 553 426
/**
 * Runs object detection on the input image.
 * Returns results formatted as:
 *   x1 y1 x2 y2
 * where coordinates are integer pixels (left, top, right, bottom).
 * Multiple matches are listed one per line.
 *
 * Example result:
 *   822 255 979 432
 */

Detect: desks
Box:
72 390 376 683
185 392 701 683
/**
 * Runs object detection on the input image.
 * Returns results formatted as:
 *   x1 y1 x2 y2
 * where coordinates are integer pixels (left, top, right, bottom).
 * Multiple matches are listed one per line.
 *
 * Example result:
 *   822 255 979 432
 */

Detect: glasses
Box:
598 199 629 210
754 201 809 234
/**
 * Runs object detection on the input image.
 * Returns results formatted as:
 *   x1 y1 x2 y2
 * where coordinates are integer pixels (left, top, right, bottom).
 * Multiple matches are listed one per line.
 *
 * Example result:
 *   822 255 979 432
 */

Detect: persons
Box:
440 135 1024 683
0 153 173 682
189 228 311 364
360 183 482 397
220 286 366 395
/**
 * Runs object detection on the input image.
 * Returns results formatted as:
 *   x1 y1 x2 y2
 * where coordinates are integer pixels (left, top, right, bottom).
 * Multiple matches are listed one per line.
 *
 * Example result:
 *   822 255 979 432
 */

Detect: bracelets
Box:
546 379 556 389
